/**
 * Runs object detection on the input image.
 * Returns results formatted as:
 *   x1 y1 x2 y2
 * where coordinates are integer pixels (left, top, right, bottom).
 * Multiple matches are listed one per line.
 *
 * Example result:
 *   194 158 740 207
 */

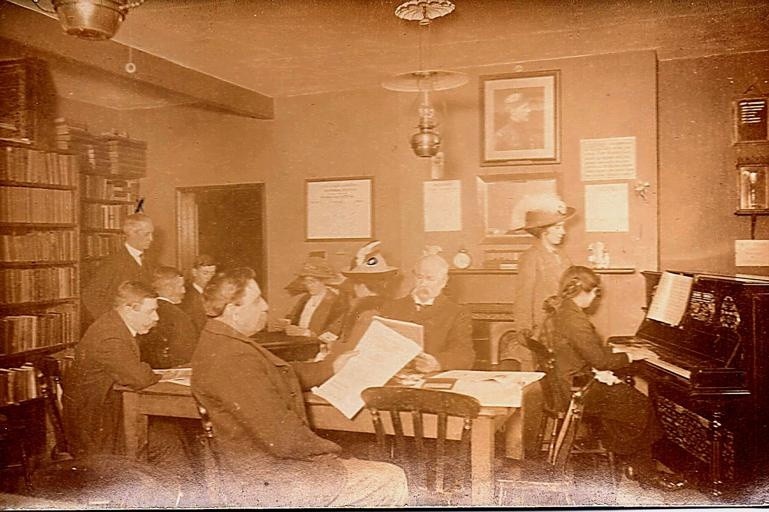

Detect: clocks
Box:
452 249 472 269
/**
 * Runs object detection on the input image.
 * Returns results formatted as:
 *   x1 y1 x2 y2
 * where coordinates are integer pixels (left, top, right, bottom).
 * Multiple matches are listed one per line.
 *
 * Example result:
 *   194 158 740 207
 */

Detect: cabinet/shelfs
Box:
0 140 148 451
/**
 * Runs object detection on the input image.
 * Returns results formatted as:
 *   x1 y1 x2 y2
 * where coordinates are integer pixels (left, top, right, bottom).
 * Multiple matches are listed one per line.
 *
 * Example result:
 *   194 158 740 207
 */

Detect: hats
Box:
341 248 399 275
502 94 534 113
297 256 336 277
511 203 577 232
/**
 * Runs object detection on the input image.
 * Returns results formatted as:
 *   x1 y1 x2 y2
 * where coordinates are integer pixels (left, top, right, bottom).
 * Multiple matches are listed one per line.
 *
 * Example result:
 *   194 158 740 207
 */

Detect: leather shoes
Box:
627 464 689 491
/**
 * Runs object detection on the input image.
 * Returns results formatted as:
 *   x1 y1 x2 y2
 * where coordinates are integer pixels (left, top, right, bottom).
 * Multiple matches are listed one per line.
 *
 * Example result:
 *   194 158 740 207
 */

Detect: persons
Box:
491 91 542 151
271 239 477 377
512 194 579 371
64 281 197 485
61 215 481 506
543 264 690 490
78 217 216 369
193 269 409 507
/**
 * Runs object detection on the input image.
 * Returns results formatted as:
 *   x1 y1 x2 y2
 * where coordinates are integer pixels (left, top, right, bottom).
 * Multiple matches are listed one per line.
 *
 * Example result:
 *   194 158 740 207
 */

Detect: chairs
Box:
36 356 68 460
362 387 481 508
192 394 223 475
525 337 618 492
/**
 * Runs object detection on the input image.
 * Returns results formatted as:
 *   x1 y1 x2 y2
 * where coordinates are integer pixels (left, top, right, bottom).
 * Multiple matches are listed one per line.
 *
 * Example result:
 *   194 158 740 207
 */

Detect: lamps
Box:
33 0 147 41
381 0 471 158
733 157 769 215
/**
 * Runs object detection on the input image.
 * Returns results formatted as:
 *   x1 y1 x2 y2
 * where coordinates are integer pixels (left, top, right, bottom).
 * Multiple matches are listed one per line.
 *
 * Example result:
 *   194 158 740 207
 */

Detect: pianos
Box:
608 269 768 504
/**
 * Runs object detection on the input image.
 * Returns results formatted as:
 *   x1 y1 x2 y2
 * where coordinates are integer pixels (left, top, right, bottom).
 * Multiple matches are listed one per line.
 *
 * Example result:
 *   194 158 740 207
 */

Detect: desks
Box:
253 331 318 350
114 363 525 506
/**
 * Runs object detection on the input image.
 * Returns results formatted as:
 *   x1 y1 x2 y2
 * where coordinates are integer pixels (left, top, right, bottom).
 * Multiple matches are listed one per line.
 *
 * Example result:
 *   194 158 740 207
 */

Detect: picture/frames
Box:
479 69 561 167
303 176 375 242
476 172 562 245
734 96 769 144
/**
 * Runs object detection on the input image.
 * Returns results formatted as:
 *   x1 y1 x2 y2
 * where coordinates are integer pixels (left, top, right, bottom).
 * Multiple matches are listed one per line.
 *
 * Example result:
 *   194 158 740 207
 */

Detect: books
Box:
646 273 695 327
3 56 146 401
61 216 125 375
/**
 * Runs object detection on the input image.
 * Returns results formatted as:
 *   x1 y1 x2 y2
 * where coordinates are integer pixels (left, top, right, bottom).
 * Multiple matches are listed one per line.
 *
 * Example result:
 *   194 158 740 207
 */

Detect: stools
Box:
0 418 34 490
